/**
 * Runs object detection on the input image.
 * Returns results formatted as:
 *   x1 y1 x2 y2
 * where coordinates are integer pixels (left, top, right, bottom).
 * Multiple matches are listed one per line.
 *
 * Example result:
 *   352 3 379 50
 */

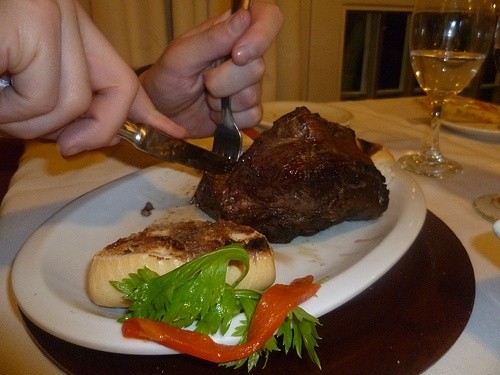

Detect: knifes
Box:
116 115 235 175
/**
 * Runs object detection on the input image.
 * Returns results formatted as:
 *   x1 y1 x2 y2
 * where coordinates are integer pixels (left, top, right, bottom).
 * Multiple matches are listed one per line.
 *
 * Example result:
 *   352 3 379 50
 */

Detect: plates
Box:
11 156 427 357
438 119 500 141
259 102 354 128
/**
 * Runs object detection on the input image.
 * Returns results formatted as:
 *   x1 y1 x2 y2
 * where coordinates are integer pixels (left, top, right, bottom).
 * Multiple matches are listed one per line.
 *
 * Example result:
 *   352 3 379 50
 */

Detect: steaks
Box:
191 106 391 243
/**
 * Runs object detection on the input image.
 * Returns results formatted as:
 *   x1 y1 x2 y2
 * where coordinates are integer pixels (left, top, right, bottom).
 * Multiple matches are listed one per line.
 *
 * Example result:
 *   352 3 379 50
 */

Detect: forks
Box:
210 0 251 161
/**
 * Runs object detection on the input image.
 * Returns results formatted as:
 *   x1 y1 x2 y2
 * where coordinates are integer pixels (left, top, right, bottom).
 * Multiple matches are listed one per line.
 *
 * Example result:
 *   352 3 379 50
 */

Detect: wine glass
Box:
473 192 500 222
398 0 496 180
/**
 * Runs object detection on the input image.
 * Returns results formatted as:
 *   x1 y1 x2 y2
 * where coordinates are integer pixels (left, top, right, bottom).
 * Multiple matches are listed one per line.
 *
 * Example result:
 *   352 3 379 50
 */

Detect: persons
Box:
0 1 285 158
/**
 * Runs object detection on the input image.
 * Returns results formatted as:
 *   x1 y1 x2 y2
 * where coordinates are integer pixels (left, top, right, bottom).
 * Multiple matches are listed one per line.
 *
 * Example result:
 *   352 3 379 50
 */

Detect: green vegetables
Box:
106 243 323 373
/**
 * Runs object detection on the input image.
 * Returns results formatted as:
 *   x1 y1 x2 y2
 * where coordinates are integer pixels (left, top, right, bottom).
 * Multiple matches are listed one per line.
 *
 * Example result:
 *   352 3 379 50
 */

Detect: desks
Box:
0 96 500 375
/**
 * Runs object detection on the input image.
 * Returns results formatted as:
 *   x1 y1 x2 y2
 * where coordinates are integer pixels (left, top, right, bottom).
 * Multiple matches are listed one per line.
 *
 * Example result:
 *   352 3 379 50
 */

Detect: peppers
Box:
119 273 322 363
